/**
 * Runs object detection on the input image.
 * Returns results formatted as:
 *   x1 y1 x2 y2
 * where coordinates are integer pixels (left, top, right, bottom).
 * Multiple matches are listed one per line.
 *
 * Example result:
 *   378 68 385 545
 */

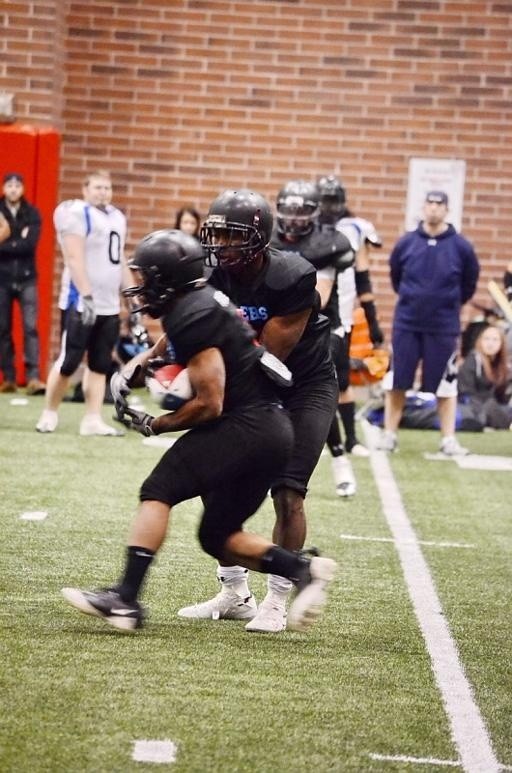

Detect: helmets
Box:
316 175 345 213
120 227 207 319
275 180 322 235
199 189 273 269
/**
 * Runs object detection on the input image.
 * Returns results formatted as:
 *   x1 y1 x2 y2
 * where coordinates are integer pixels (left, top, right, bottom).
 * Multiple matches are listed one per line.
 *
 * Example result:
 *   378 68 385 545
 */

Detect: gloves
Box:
370 327 383 343
122 285 141 323
113 407 155 437
109 370 131 411
81 294 97 326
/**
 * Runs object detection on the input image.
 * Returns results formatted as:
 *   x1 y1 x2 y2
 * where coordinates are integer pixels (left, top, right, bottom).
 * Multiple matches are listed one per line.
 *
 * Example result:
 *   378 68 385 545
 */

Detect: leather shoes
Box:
26 379 45 394
0 380 17 392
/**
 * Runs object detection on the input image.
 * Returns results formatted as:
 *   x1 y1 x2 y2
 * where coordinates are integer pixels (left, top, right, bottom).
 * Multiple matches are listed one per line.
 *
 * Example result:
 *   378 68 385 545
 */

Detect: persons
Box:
62 176 384 636
376 191 481 458
0 171 47 396
35 170 126 438
457 327 512 431
0 212 11 244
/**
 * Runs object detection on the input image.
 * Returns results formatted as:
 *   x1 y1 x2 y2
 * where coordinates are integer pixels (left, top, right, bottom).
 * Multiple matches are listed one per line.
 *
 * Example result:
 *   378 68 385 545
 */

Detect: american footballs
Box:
151 365 194 409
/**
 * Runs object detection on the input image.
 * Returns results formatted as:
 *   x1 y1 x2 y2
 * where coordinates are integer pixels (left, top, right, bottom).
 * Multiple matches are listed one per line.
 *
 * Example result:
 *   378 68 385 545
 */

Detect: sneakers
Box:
245 600 287 632
177 590 258 619
346 438 370 457
79 418 126 436
376 429 398 451
439 438 470 456
35 409 57 432
331 454 356 495
59 586 140 629
287 554 339 631
